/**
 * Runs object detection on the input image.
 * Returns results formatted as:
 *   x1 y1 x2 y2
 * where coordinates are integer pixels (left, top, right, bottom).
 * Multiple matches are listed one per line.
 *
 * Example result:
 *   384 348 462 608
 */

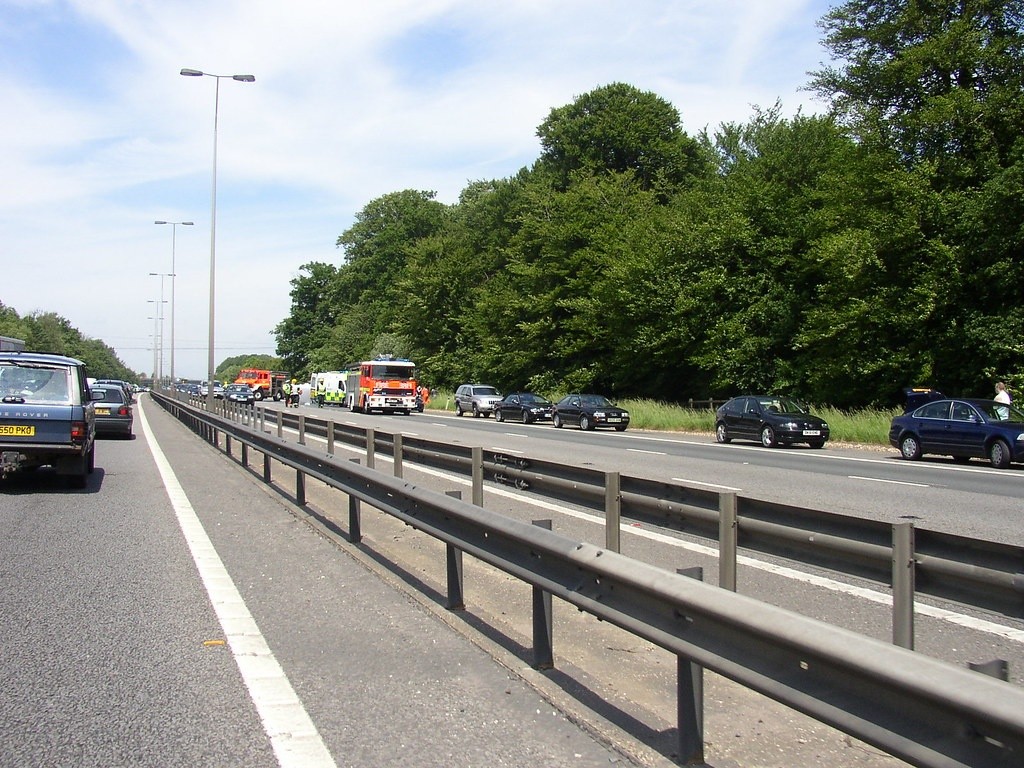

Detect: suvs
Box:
223 384 255 406
0 349 97 482
199 380 225 400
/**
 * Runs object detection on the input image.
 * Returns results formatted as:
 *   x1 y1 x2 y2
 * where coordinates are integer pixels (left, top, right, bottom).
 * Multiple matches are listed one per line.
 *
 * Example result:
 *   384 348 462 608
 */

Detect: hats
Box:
285 379 290 382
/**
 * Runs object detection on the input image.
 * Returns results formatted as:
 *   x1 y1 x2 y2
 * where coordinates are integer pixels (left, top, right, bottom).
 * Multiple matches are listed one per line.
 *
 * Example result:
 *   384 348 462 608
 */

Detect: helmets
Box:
319 378 324 381
292 378 297 384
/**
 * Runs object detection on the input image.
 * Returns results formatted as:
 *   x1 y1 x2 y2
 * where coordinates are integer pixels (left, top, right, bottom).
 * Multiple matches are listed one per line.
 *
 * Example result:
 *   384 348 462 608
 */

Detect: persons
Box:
993 382 1010 420
283 379 301 408
417 385 438 407
316 379 326 407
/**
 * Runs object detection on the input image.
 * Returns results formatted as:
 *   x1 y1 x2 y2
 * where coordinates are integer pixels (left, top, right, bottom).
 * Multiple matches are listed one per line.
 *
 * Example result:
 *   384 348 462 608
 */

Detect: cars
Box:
453 383 507 418
552 396 630 433
86 377 151 405
165 382 202 399
86 384 135 438
492 393 559 425
713 394 830 449
888 396 1024 469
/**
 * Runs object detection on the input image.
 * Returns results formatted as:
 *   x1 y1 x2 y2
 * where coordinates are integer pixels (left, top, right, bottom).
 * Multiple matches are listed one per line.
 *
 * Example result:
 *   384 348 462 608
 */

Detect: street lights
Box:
179 69 257 411
148 272 176 387
146 299 168 384
154 219 195 387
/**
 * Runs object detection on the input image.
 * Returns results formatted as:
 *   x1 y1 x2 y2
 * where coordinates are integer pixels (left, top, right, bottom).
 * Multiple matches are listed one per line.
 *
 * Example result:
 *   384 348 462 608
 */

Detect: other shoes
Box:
321 405 323 408
295 405 298 408
290 405 292 408
318 405 320 408
286 403 288 407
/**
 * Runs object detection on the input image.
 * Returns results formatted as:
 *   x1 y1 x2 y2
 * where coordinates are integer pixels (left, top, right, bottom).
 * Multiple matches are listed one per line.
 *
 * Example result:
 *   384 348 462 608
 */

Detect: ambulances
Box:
310 370 362 408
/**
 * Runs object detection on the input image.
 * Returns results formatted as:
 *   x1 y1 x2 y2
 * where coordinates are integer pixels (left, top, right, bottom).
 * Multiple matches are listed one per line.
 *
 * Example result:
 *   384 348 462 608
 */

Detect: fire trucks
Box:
234 369 292 402
345 354 417 416
